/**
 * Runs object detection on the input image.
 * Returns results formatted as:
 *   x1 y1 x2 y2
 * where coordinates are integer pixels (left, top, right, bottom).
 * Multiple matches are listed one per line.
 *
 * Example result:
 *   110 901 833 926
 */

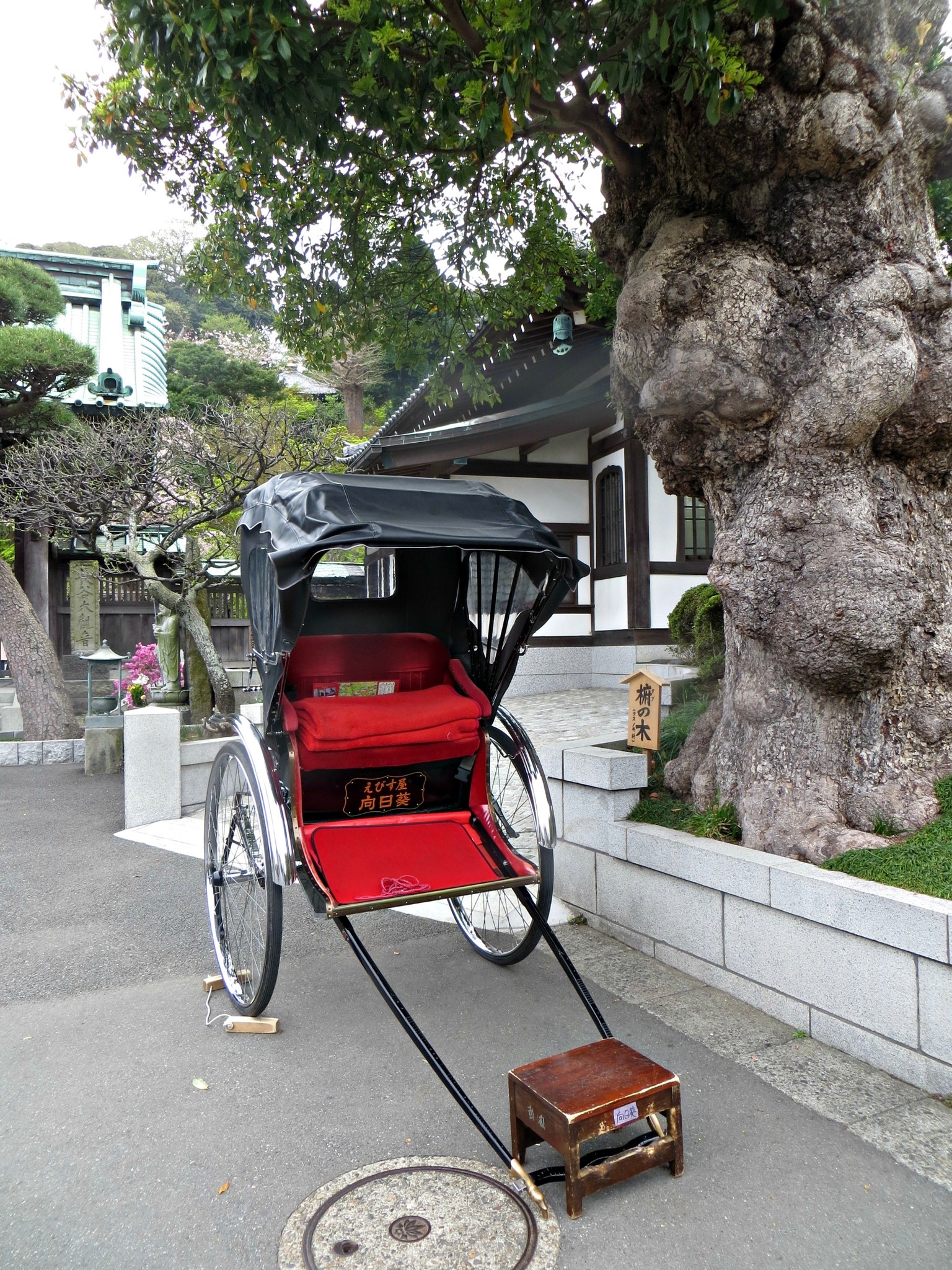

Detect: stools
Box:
507 1041 684 1222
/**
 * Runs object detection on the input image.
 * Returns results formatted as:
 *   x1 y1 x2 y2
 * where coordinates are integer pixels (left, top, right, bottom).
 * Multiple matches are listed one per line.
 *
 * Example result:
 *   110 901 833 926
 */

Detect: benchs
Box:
279 633 493 824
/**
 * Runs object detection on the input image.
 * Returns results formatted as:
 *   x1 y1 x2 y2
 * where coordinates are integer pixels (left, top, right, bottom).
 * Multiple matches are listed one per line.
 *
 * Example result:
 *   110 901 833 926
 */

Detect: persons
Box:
154 602 168 626
152 602 181 691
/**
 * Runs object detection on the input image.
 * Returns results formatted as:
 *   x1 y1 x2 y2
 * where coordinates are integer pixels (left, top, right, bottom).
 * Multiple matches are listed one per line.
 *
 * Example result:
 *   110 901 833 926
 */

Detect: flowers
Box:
115 641 186 707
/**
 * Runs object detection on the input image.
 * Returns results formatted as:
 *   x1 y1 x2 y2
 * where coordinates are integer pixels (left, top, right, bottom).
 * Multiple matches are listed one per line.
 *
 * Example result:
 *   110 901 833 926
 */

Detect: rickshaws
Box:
198 475 670 1182
